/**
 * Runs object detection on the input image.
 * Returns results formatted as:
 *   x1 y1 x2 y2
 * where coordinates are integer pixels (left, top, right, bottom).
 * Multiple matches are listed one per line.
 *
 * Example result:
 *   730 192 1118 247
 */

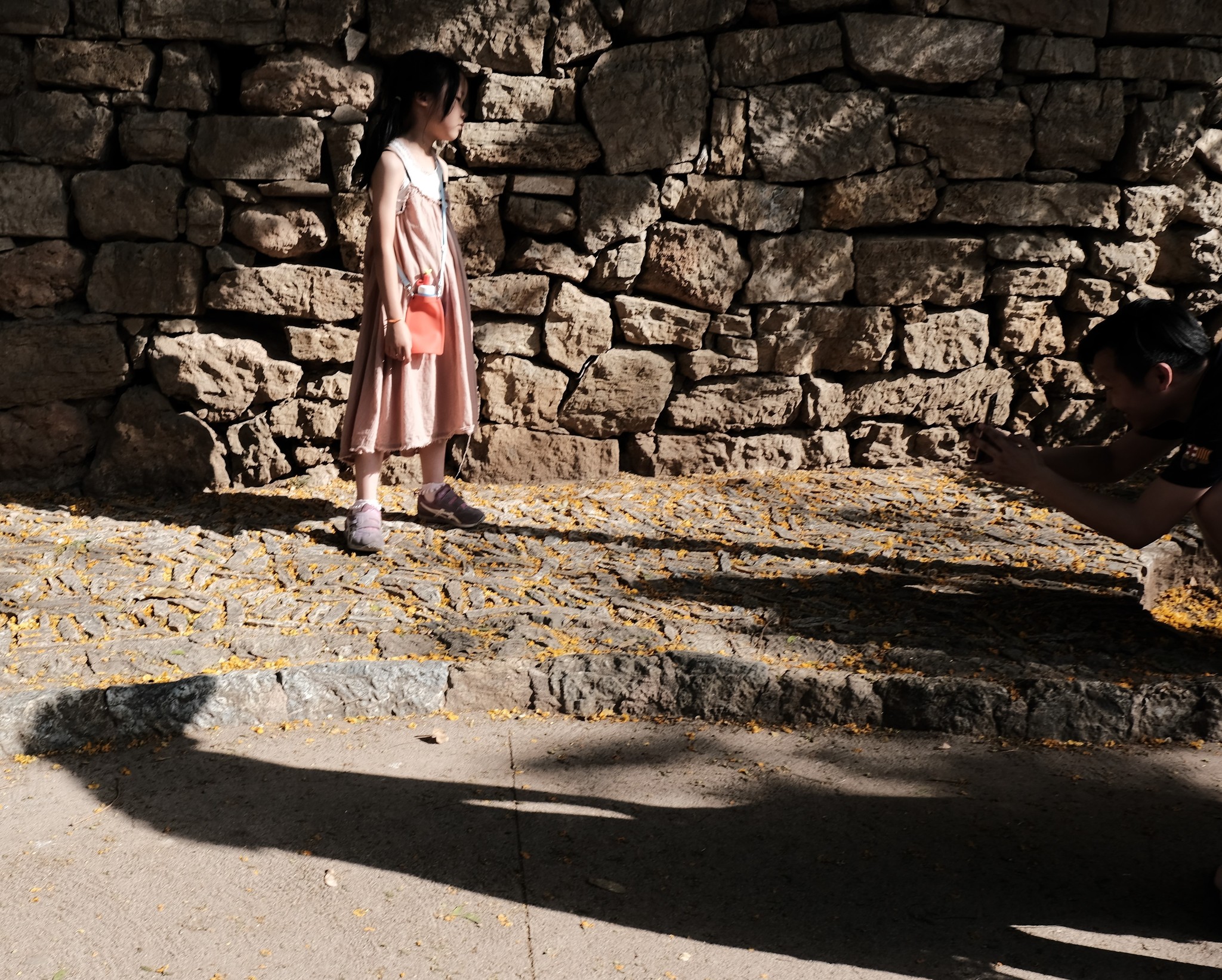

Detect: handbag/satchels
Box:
404 293 445 356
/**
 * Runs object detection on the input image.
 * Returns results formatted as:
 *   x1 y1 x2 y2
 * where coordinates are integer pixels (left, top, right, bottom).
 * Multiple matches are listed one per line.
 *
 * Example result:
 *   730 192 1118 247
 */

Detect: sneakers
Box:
344 504 385 553
417 483 486 529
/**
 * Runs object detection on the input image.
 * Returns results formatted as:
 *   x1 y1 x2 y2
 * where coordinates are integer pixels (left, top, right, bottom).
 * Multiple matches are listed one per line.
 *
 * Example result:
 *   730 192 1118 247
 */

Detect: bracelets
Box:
388 318 405 323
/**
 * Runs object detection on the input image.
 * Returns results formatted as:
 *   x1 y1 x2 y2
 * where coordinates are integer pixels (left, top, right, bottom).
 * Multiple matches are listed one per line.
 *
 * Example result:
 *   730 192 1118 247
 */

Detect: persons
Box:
338 48 486 553
967 299 1222 566
